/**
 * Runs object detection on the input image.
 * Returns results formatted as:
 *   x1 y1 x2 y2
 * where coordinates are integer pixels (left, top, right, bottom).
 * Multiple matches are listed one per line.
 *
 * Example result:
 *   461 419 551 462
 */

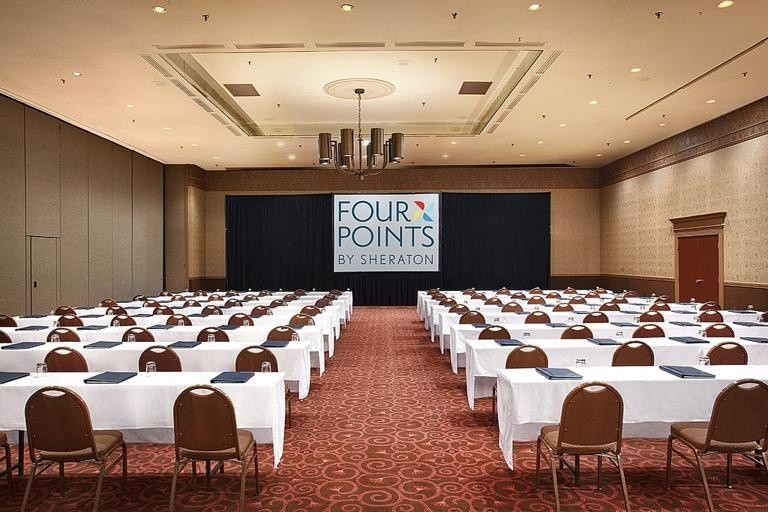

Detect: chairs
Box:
416 287 767 512
0 286 359 512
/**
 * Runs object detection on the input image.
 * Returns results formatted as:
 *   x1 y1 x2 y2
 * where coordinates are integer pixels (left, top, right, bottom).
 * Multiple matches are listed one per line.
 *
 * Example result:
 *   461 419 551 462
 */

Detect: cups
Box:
127 334 135 344
144 362 157 379
698 357 712 368
434 284 762 344
34 362 48 379
576 357 588 367
47 287 350 328
260 361 271 378
51 333 59 345
291 332 300 345
207 334 215 344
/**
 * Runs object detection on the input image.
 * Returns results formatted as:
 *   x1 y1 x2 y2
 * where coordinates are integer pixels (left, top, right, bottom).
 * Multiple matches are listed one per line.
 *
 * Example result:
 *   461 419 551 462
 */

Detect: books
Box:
83 372 136 385
668 336 708 344
14 294 304 332
587 338 622 345
534 367 581 380
0 372 28 384
83 341 121 349
739 337 767 343
455 296 767 329
166 341 200 349
260 341 288 347
494 338 523 346
210 371 255 385
1 342 45 350
658 366 716 379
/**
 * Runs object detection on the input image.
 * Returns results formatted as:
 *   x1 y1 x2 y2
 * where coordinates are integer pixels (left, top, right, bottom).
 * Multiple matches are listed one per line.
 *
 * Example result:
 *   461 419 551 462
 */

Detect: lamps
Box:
318 87 405 177
